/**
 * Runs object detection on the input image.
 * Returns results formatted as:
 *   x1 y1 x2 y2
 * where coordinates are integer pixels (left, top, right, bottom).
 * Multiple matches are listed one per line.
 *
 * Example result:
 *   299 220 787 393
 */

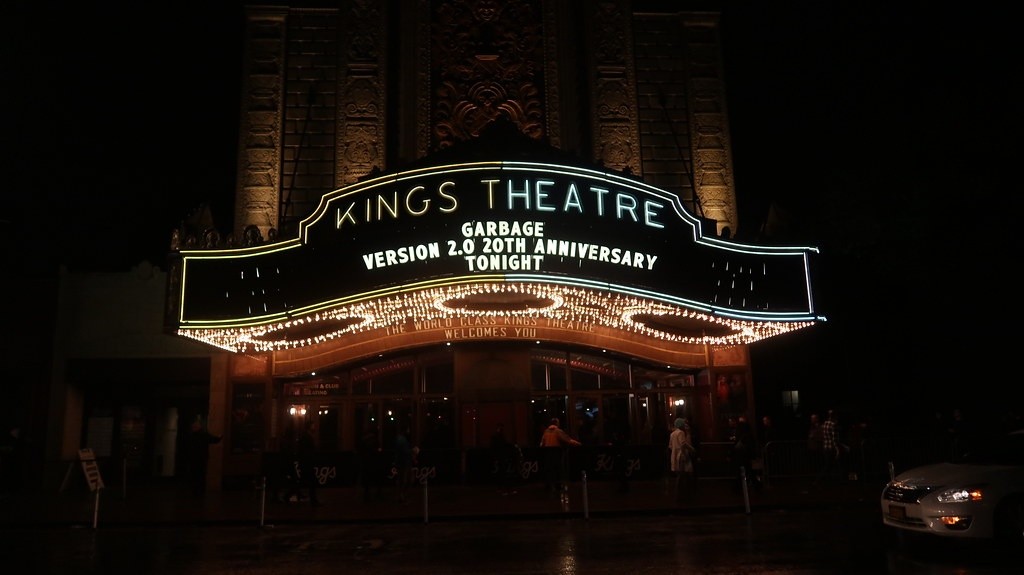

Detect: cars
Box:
879 426 1024 564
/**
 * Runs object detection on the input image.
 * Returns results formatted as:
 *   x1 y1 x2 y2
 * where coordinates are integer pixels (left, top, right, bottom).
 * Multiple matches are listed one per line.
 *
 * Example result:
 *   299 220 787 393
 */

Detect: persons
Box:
540 418 581 490
175 420 223 498
488 423 518 483
390 421 420 506
668 410 842 495
277 420 323 506
